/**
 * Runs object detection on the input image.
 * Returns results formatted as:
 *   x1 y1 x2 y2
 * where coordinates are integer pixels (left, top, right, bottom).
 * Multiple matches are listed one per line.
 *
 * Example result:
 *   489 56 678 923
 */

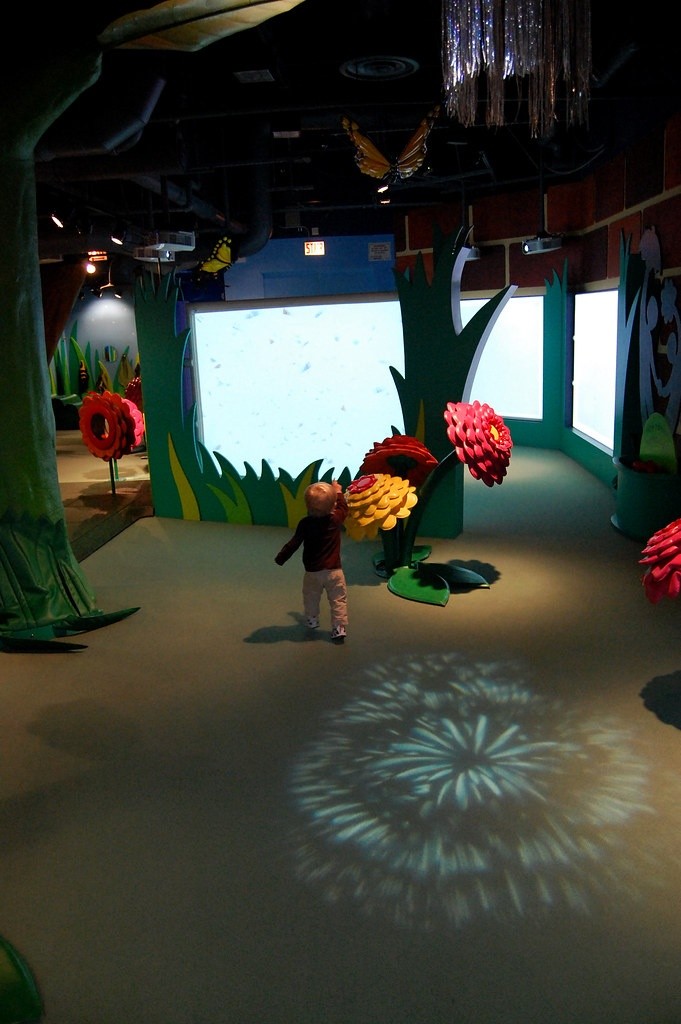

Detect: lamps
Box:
111 222 128 246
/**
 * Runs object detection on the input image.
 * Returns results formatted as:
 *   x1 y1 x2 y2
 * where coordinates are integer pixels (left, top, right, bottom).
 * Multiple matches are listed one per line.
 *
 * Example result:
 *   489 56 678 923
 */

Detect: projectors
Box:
522 238 562 255
133 247 176 263
143 231 197 252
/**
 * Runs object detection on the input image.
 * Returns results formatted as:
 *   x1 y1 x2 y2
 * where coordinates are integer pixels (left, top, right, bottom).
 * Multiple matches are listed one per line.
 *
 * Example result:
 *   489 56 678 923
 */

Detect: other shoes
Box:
330 625 347 639
304 615 320 629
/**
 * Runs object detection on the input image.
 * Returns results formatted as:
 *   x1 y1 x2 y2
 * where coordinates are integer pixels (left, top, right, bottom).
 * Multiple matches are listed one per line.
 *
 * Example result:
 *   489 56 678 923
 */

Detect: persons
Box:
274 478 350 639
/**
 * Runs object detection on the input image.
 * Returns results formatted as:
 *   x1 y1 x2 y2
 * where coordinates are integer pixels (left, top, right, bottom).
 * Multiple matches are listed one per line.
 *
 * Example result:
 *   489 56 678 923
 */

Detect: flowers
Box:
639 520 681 604
79 377 145 504
340 399 514 606
